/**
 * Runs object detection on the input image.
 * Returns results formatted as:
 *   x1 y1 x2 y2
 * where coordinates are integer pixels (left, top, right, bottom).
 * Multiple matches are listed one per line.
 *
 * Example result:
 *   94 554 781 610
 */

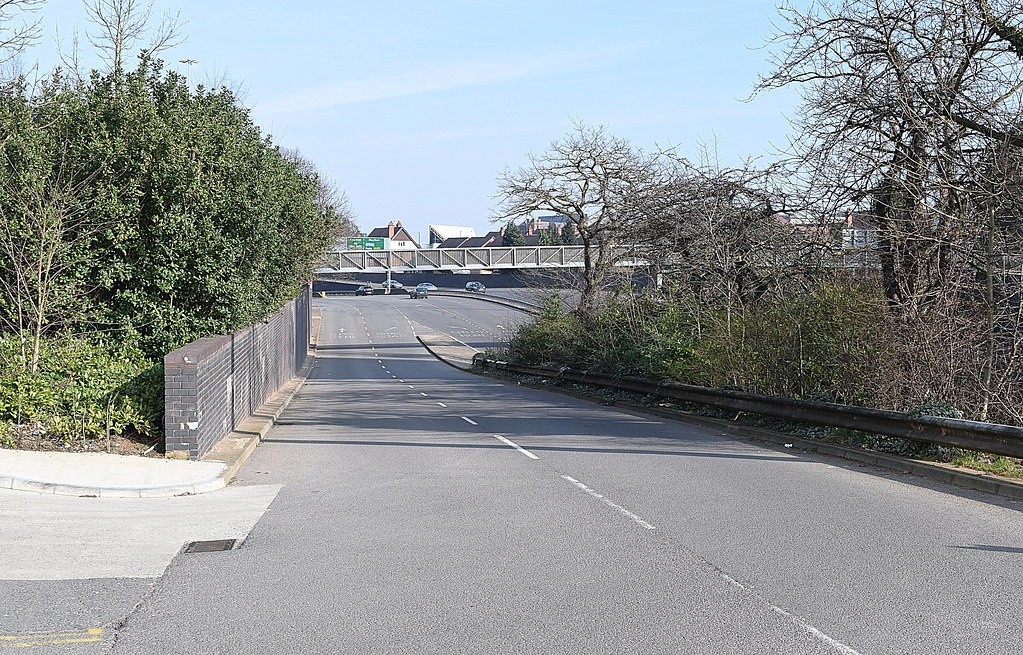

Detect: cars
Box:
409 288 428 299
416 282 438 292
382 280 403 288
466 281 486 293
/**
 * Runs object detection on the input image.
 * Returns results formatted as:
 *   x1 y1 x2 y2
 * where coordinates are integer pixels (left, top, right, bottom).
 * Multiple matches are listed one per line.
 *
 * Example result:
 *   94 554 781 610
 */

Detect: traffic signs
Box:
346 237 384 251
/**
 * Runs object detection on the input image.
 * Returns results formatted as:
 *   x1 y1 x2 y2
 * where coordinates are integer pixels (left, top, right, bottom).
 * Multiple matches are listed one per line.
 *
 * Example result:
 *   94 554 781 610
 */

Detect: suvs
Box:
356 287 374 295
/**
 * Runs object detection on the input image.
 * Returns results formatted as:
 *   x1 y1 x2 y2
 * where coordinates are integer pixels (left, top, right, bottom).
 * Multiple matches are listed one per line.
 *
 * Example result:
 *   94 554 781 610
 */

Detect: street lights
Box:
418 232 420 245
